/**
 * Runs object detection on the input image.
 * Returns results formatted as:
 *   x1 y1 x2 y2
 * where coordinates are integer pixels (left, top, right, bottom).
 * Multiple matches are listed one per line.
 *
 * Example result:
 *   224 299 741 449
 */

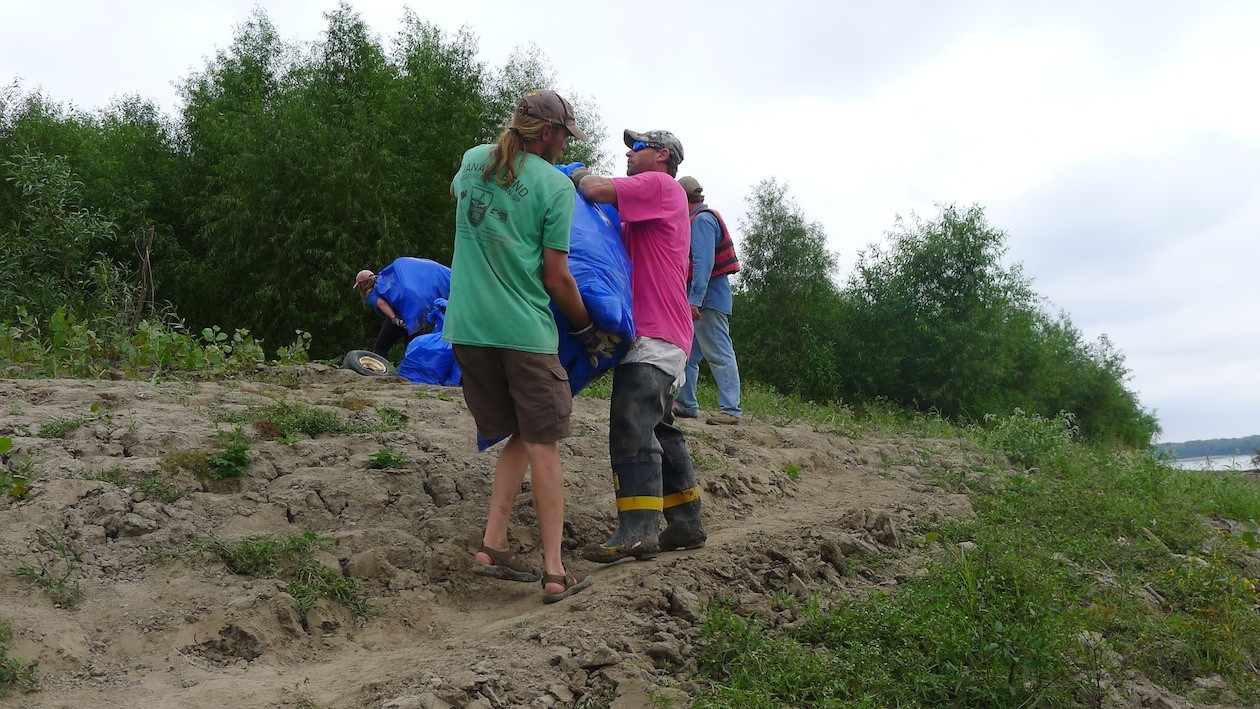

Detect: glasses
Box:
632 140 663 152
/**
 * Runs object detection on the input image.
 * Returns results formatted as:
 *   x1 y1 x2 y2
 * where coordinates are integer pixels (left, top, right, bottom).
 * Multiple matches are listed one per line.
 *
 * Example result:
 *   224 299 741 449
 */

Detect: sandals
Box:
540 563 594 603
471 541 541 582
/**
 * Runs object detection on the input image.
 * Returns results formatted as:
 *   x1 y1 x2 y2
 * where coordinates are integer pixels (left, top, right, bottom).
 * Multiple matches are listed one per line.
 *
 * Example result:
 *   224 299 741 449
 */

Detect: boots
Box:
583 462 663 564
658 457 707 550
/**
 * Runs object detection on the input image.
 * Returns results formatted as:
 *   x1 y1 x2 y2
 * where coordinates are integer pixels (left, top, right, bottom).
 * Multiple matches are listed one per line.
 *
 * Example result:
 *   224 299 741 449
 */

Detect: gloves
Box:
567 321 622 369
571 166 590 188
392 315 406 328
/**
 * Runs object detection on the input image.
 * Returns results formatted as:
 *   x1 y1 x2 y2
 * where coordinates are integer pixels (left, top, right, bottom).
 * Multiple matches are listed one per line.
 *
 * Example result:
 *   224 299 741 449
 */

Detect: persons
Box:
671 175 742 426
352 270 424 360
553 129 707 564
440 89 621 604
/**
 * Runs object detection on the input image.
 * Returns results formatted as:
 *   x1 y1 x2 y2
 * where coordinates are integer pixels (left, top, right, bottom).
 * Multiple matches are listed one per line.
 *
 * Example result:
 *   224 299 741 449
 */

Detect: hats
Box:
624 128 684 165
517 89 589 141
353 270 374 288
678 175 704 202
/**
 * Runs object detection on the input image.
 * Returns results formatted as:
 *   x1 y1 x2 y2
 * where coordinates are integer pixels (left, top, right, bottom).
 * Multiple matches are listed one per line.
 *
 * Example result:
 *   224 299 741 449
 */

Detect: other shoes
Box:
671 407 698 418
707 412 740 425
